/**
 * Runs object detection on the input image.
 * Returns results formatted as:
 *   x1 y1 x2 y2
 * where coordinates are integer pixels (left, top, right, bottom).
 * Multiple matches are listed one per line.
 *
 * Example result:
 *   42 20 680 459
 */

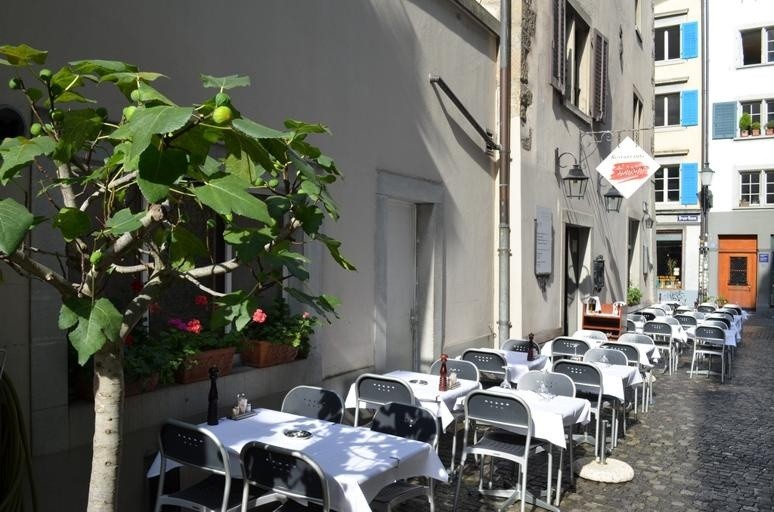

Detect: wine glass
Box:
500 366 511 389
599 346 609 368
570 344 580 362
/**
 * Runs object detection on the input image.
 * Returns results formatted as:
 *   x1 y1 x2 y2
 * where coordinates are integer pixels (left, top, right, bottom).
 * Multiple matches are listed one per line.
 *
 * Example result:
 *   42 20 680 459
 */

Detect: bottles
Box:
448 369 458 388
613 303 618 315
533 348 538 358
230 393 252 417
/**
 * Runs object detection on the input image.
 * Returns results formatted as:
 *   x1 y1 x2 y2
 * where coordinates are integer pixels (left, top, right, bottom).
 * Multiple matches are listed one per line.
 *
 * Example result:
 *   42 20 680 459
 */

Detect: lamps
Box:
598 175 623 213
642 212 654 232
556 151 590 200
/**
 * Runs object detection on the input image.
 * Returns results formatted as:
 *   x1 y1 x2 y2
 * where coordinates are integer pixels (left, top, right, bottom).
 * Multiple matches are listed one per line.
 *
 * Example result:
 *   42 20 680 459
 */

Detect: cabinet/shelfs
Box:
582 303 622 339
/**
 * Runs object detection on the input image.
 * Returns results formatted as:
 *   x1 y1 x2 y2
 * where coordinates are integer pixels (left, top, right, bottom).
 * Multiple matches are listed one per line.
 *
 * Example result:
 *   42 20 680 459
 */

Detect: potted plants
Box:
738 113 751 137
764 119 774 135
666 256 677 286
751 121 760 136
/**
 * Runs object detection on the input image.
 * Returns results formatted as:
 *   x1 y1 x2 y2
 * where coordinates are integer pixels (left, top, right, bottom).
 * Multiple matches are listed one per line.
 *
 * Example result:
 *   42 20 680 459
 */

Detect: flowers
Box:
170 294 239 368
241 297 317 359
123 281 171 371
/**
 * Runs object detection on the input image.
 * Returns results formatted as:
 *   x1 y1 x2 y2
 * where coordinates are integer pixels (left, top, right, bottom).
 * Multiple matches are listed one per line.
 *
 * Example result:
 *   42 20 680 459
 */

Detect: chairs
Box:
239 441 331 512
627 302 743 384
281 385 344 424
154 417 241 512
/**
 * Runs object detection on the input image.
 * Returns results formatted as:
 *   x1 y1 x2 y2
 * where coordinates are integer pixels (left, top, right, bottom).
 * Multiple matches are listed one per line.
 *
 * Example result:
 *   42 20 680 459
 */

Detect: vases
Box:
240 339 298 367
124 370 161 396
174 347 236 384
